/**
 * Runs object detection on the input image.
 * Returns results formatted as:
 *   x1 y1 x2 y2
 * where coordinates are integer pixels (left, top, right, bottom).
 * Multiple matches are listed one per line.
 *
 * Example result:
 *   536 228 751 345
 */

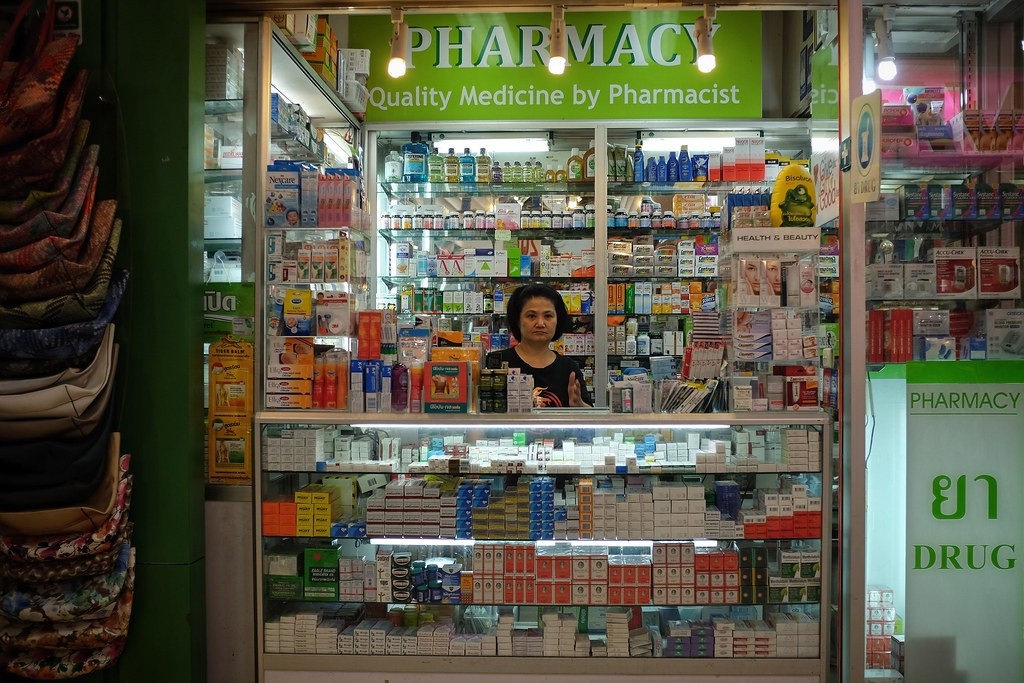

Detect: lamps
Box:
693 3 720 74
549 0 567 74
874 2 901 82
385 4 409 78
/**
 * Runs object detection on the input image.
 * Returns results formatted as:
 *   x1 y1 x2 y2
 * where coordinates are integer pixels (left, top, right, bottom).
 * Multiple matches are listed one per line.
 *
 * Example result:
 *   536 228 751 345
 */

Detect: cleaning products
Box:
382 132 595 179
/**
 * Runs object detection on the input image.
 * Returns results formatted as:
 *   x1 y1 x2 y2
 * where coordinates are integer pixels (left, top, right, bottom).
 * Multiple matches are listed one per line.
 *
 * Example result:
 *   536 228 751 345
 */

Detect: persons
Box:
481 282 595 444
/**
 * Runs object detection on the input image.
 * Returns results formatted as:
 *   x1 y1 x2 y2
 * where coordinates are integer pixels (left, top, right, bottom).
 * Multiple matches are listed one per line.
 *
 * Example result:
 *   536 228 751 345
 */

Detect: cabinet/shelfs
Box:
868 115 1021 366
255 413 828 666
206 25 372 356
372 123 601 407
606 121 840 408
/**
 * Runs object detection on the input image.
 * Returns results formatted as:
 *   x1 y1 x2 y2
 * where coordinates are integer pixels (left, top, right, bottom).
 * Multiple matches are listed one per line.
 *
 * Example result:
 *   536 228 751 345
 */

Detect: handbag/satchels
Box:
0 0 139 683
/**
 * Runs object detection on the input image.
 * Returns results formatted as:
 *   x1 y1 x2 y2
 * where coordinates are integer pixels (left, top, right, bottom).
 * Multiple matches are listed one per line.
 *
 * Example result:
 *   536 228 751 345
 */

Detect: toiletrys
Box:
633 137 766 182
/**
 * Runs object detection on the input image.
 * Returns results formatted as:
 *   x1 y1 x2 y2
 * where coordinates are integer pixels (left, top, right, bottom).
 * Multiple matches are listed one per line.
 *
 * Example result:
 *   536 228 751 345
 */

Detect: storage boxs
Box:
257 92 1023 657
